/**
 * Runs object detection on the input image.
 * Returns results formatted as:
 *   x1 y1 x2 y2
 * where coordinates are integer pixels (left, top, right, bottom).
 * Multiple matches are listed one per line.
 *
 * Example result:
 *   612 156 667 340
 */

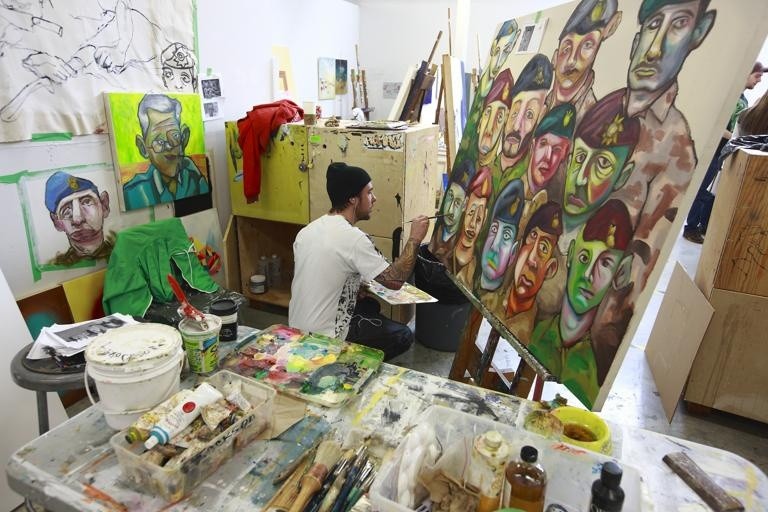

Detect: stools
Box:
9 341 98 437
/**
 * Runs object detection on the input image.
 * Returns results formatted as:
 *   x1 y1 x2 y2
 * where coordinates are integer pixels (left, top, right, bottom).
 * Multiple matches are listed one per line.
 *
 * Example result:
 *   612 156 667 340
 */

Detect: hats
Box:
326 162 370 208
752 62 768 73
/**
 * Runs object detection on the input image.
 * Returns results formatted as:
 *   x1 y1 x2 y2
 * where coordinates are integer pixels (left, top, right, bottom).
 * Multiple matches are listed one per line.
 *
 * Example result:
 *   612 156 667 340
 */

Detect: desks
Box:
6 324 767 511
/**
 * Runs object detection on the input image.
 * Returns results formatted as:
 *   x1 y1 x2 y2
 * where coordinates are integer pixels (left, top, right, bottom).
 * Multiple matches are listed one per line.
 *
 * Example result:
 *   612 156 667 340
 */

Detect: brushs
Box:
167 274 209 332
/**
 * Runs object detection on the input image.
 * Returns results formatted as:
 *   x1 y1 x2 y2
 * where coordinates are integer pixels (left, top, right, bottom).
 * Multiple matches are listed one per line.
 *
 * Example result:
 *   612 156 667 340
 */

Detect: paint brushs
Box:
402 215 448 223
273 428 378 512
351 43 368 111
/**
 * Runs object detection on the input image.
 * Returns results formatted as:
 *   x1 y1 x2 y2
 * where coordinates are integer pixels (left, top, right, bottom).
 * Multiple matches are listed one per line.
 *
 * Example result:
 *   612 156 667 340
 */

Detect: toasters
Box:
589 460 625 511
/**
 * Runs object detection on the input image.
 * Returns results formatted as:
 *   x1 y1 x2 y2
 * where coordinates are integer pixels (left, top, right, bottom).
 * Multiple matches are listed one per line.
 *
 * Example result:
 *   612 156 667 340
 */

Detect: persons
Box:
682 61 768 244
287 161 432 362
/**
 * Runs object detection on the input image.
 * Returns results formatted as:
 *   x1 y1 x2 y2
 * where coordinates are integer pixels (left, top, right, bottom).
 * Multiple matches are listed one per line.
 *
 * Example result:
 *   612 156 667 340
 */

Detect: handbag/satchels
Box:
719 135 768 161
692 189 715 219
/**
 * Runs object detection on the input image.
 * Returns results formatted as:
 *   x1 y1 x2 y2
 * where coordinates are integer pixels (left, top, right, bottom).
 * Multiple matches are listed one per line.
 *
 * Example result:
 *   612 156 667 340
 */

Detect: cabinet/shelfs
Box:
682 148 768 425
224 117 441 326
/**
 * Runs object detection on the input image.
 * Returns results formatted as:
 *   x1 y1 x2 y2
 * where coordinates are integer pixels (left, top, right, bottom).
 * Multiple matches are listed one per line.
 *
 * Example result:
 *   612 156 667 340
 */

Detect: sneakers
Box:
683 225 704 244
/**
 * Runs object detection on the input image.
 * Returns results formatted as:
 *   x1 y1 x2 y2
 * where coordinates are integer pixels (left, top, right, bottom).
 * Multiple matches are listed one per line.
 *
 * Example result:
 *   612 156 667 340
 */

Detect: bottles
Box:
466 429 506 512
501 444 548 512
257 254 281 289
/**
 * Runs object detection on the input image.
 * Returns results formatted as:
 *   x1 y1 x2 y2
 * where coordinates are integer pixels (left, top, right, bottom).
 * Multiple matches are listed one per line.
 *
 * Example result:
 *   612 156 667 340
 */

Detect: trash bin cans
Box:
415 242 474 352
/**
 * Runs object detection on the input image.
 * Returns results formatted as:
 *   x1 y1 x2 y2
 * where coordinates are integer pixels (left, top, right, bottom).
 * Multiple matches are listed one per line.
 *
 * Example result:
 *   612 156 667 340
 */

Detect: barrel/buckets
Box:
179 314 222 374
84 323 185 430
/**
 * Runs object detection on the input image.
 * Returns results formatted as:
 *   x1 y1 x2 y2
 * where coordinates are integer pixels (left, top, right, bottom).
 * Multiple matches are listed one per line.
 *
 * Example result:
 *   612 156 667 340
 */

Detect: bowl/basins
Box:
547 406 610 454
